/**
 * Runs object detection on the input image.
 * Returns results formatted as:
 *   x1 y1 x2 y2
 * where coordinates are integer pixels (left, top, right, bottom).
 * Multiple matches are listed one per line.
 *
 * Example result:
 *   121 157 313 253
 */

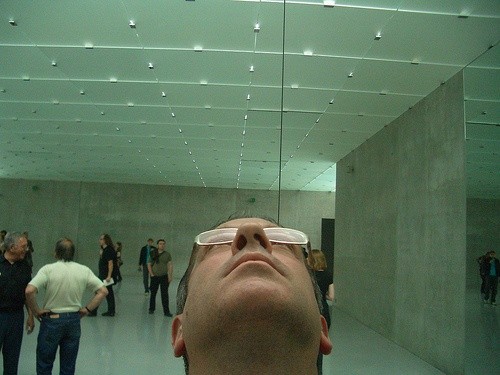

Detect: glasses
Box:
192 227 311 258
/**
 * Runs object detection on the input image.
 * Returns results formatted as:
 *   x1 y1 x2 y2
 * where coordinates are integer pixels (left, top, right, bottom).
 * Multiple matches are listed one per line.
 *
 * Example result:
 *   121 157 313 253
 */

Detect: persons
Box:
170 212 332 375
21 231 34 282
86 233 117 316
478 250 500 305
24 239 109 375
0 232 35 375
147 239 173 317
114 241 124 283
139 238 156 296
305 249 335 336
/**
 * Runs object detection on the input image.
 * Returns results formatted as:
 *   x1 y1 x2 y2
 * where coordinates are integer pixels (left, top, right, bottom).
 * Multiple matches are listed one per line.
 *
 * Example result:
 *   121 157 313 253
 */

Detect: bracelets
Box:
85 306 92 313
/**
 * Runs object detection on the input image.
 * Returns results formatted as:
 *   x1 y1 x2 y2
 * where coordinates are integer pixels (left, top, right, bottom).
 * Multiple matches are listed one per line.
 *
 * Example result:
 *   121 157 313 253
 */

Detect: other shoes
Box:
149 311 154 314
144 292 148 296
102 312 115 316
164 314 174 317
